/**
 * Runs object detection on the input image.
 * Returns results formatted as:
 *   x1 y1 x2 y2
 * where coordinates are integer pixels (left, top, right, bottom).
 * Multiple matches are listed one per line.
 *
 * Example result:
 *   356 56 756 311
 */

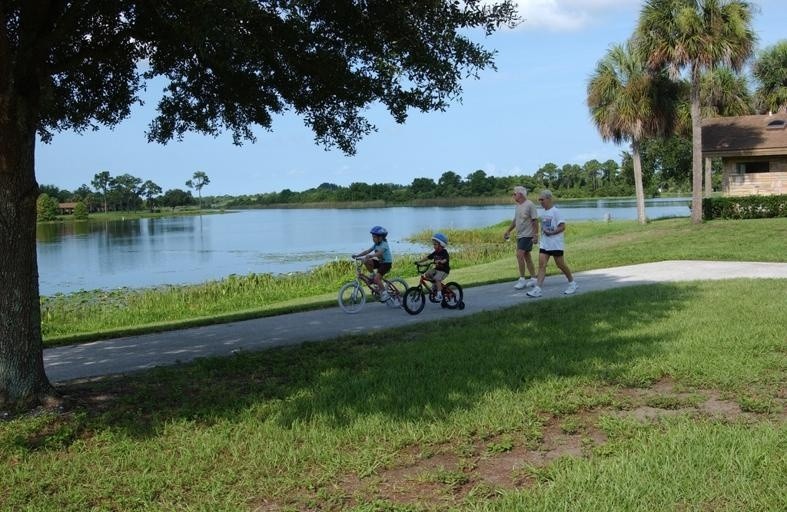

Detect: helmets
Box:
370 226 388 236
432 233 448 247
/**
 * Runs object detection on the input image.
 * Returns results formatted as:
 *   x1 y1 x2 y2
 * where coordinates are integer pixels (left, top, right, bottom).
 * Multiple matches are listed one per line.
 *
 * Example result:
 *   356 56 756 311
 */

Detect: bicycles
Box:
402 262 465 315
338 257 409 314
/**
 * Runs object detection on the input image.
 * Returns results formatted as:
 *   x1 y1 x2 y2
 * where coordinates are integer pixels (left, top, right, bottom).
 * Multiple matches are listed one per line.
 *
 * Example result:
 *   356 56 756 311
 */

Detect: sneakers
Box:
435 295 442 300
565 282 579 293
513 278 537 289
366 275 375 283
380 294 390 302
527 288 544 297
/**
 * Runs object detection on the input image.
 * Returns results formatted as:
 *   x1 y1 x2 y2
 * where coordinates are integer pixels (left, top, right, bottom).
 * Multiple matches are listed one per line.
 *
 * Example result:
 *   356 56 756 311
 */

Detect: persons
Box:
526 189 577 298
414 233 450 301
352 225 393 303
504 185 540 290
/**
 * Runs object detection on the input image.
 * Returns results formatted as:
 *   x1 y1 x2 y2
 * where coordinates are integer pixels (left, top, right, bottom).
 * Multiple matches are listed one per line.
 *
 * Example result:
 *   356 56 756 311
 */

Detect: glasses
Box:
512 194 516 196
539 199 544 201
